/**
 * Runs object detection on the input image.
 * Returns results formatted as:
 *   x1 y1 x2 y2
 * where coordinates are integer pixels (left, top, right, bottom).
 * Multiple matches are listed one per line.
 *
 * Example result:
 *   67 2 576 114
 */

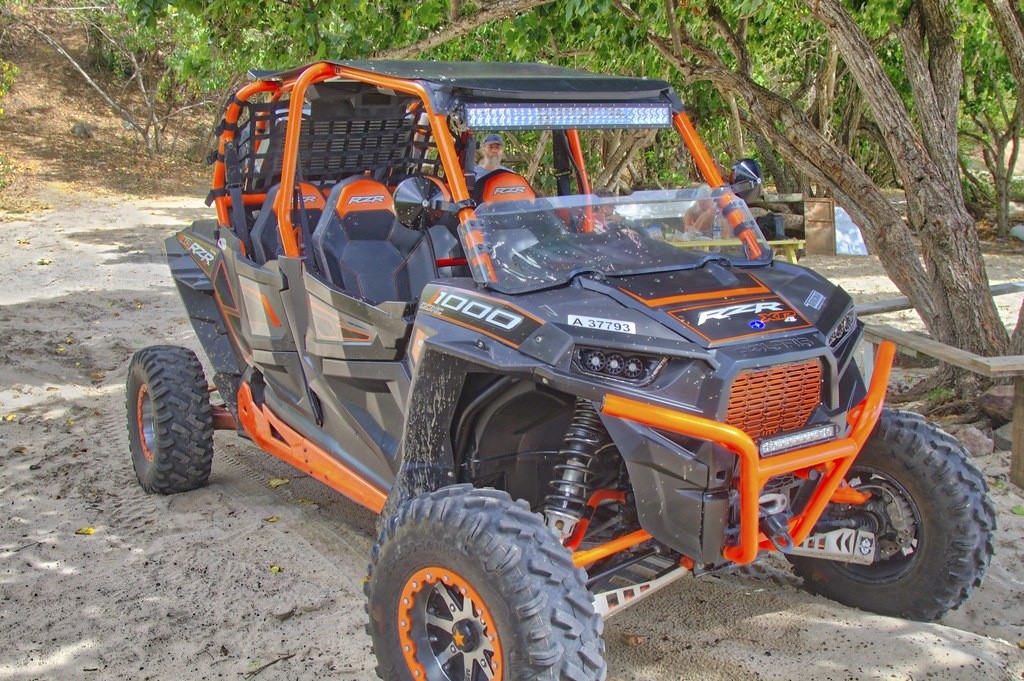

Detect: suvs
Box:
122 66 1004 680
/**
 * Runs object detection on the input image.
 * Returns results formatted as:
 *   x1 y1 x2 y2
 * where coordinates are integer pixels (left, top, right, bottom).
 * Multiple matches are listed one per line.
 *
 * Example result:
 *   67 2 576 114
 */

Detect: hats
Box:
482 133 503 145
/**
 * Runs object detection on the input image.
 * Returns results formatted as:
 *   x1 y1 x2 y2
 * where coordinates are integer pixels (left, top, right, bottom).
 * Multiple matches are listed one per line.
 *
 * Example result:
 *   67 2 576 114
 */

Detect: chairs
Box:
252 169 571 306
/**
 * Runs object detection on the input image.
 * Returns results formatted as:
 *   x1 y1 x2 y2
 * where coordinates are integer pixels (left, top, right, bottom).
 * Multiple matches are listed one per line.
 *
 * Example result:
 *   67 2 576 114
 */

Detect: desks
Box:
667 240 806 266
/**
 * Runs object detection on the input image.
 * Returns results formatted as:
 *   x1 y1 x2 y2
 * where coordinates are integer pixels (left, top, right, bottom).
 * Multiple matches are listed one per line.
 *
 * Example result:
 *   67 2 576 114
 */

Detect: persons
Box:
684 185 717 234
474 134 516 183
